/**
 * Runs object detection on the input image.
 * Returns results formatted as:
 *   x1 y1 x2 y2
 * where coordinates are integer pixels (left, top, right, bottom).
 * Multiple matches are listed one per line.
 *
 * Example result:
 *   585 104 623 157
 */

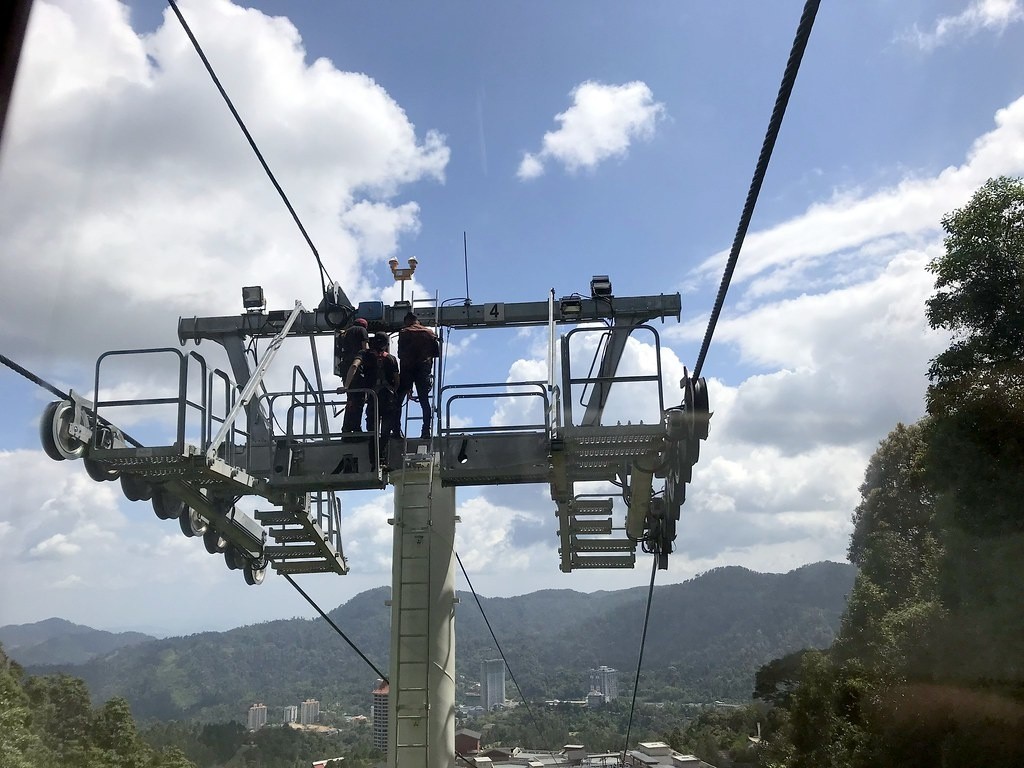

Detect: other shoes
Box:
341 430 368 442
421 428 431 439
370 463 376 473
380 457 387 467
391 429 401 439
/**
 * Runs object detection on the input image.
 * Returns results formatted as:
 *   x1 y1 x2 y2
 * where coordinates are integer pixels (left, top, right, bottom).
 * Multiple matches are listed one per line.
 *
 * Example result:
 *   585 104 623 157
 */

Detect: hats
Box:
356 318 368 327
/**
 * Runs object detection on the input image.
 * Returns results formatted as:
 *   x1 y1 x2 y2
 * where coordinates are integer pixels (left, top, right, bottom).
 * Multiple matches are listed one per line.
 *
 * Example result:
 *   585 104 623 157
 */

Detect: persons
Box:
390 311 433 441
336 332 400 471
338 318 369 441
